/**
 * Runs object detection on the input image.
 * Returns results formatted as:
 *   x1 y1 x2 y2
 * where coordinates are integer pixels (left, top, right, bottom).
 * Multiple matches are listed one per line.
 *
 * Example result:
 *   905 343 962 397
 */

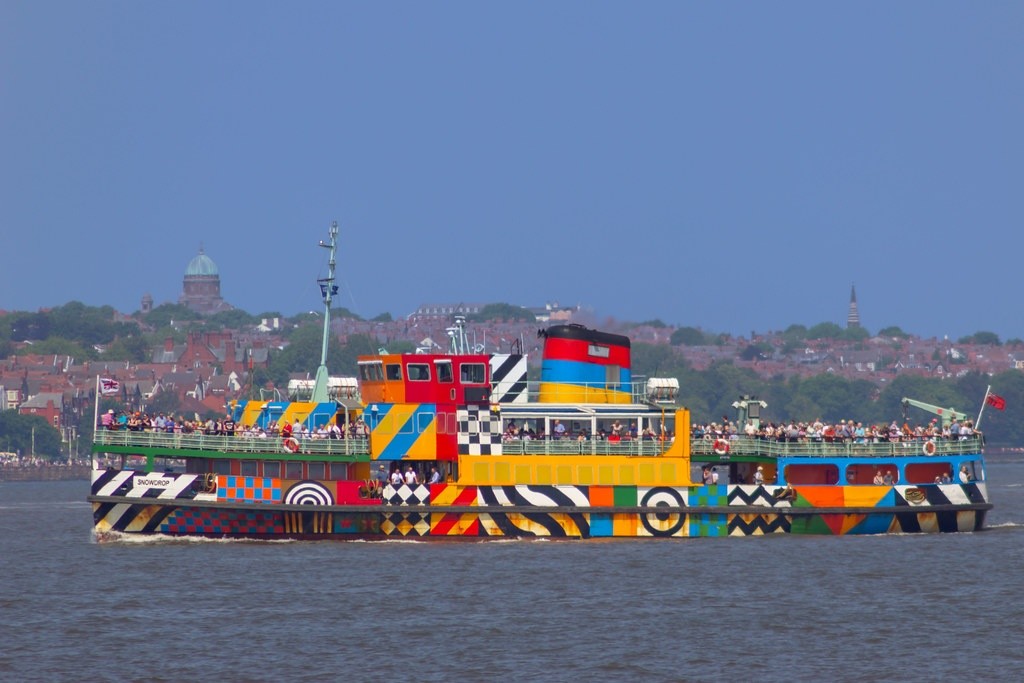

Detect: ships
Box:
87 219 1009 542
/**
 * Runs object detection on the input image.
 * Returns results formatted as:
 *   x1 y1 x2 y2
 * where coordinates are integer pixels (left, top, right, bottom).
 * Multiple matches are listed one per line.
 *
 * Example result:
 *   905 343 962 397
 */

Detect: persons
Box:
98 407 983 490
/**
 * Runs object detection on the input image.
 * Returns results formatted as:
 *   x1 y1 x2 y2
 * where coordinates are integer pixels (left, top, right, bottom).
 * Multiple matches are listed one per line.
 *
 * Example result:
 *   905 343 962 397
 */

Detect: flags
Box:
985 391 1006 410
99 378 119 394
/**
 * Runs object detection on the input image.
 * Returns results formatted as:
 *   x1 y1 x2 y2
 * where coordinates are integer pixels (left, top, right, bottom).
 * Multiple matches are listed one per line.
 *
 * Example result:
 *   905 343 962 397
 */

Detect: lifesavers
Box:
713 438 730 456
922 440 937 457
282 437 299 454
